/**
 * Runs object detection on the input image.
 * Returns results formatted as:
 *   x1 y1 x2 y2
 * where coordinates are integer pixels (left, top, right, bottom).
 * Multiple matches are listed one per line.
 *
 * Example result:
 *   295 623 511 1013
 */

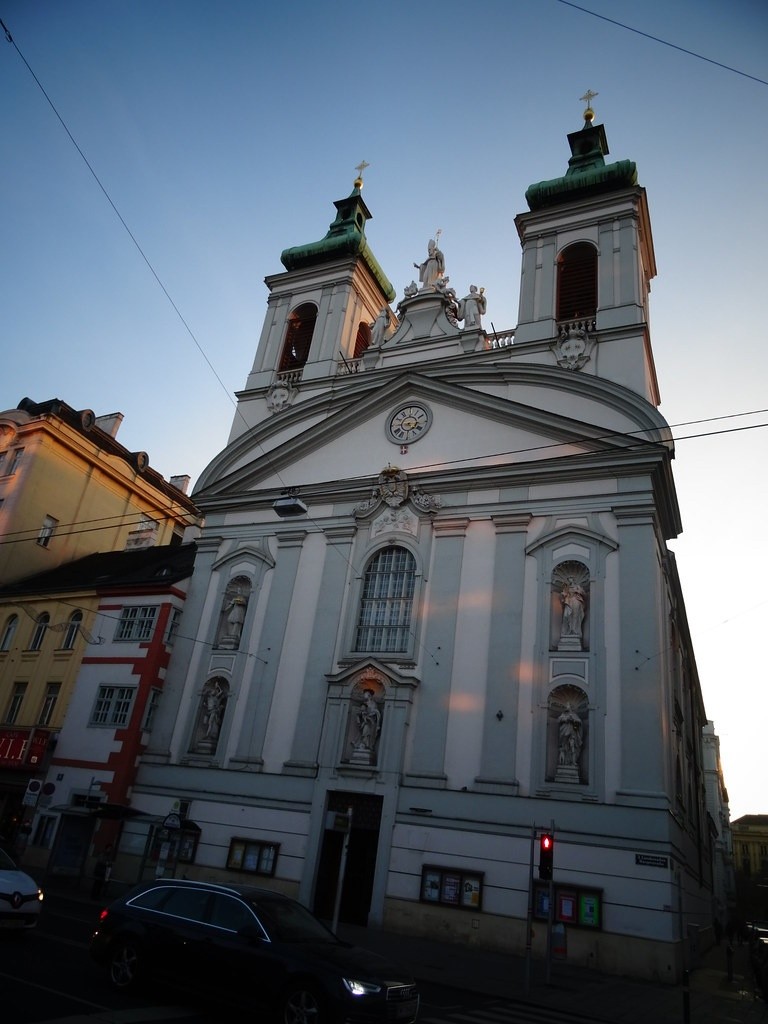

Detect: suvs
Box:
94 877 422 1024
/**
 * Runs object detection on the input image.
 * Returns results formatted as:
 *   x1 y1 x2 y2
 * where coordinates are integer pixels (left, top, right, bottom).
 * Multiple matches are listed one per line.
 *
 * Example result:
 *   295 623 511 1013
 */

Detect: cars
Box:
1 849 44 930
746 918 768 990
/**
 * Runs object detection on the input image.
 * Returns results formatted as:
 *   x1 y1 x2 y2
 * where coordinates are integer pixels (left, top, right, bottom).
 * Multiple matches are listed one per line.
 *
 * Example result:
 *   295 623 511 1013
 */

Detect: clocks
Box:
385 402 432 445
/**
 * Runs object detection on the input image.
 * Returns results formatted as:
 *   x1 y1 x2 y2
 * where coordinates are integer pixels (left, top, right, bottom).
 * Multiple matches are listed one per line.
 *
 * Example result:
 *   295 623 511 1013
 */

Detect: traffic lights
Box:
538 835 554 879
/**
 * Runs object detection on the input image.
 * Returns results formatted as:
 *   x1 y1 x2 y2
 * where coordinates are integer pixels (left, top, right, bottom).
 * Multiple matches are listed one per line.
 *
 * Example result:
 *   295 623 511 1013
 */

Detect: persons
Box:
557 702 584 766
368 305 391 349
198 680 224 740
560 574 586 637
14 819 32 855
222 586 247 636
458 285 485 326
414 240 445 286
350 687 381 749
713 916 744 945
